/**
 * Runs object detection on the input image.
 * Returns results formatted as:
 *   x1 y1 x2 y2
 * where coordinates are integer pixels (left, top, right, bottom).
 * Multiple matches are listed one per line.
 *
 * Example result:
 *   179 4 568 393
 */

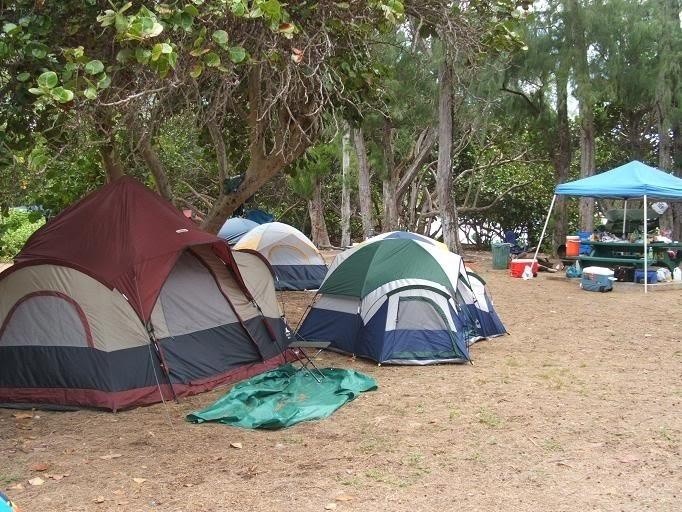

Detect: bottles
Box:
648 247 653 258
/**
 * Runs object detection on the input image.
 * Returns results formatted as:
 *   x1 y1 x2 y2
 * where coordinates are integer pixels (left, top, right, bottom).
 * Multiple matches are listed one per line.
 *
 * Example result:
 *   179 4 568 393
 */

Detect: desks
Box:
582 242 681 270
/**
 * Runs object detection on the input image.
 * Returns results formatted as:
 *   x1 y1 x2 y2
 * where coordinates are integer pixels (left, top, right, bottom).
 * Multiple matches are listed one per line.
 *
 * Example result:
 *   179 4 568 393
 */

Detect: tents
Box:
294 231 510 368
234 221 328 290
217 216 261 249
1 175 307 430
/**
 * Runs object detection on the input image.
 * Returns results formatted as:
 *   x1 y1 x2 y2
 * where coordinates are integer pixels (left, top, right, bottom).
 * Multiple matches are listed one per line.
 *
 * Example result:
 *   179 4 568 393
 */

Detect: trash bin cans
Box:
491 243 510 268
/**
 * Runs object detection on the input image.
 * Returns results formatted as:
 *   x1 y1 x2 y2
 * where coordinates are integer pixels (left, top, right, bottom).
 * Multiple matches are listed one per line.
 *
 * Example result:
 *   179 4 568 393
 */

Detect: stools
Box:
636 269 658 283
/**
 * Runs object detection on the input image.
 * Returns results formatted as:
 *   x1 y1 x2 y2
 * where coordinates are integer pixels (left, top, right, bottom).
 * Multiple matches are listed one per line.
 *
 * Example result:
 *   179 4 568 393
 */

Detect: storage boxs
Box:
612 266 634 282
509 259 538 277
581 267 617 292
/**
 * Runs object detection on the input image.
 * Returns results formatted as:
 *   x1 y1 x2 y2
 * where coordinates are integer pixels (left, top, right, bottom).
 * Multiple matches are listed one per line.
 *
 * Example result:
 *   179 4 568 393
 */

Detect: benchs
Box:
566 256 650 269
655 258 681 272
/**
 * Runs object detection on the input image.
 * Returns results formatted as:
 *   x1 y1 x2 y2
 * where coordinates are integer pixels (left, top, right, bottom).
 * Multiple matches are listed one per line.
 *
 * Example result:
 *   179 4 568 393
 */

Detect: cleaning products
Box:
648 247 654 260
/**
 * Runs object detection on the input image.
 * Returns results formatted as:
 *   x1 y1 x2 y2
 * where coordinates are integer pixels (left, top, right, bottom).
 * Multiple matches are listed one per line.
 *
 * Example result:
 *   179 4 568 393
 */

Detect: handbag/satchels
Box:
579 271 613 292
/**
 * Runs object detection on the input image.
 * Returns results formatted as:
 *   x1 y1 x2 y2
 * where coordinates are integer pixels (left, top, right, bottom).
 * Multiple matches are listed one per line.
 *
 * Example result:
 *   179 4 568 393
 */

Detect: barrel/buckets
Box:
579 232 592 254
566 236 581 256
673 267 681 281
492 243 511 269
